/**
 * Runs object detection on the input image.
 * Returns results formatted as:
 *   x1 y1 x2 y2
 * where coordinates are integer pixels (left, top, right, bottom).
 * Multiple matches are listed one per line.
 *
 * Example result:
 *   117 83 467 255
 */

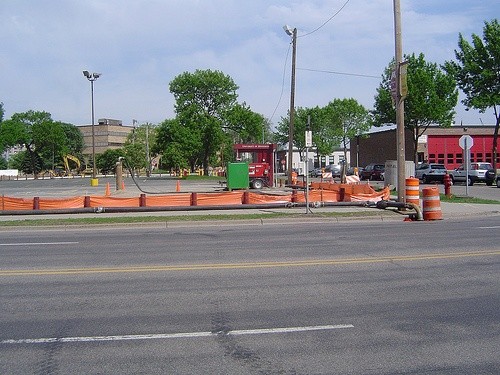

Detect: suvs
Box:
449 161 496 186
415 162 448 184
358 163 385 181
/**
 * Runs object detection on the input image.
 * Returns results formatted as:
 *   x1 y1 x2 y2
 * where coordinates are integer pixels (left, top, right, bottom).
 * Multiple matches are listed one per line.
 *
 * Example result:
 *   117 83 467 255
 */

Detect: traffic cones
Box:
176 180 181 192
122 180 124 190
104 182 110 196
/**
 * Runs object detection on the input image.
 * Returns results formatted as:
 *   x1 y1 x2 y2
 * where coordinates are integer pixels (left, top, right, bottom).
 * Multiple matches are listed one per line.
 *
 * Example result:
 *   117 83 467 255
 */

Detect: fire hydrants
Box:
443 170 453 196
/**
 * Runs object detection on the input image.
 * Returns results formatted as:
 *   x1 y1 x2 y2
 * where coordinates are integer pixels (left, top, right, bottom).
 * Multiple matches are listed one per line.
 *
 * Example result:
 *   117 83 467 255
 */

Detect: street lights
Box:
82 70 103 187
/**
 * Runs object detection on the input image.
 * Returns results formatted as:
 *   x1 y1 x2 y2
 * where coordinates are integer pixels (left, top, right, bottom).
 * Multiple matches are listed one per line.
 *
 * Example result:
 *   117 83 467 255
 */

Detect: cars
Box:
309 163 363 178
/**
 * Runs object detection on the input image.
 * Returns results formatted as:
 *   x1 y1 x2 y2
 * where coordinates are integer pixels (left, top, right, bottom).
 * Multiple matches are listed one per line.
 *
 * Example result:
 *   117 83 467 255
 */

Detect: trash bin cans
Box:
226 162 249 191
